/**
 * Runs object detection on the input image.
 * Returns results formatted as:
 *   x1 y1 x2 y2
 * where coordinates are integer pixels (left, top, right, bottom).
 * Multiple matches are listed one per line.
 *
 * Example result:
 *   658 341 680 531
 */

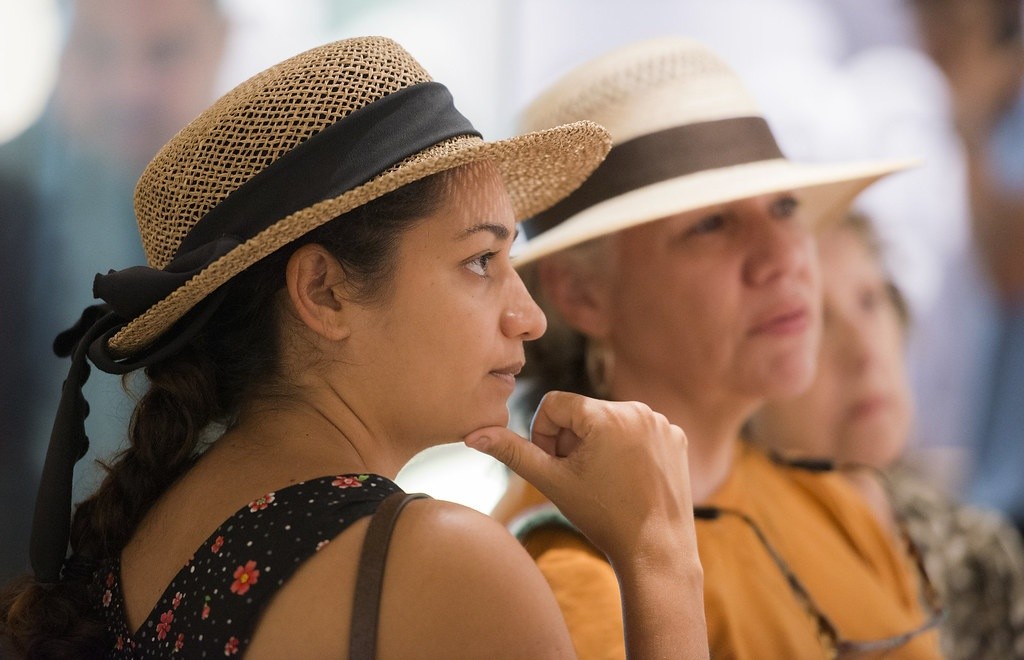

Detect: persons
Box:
0 37 714 660
0 0 258 601
470 0 1024 660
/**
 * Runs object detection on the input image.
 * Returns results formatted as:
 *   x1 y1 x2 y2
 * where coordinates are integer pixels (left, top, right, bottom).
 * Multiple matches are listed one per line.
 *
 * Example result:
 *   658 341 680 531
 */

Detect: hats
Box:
28 39 616 599
504 37 915 269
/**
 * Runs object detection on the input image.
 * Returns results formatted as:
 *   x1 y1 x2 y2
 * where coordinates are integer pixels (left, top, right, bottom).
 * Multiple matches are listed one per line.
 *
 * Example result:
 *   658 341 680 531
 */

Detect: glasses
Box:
693 449 950 660
